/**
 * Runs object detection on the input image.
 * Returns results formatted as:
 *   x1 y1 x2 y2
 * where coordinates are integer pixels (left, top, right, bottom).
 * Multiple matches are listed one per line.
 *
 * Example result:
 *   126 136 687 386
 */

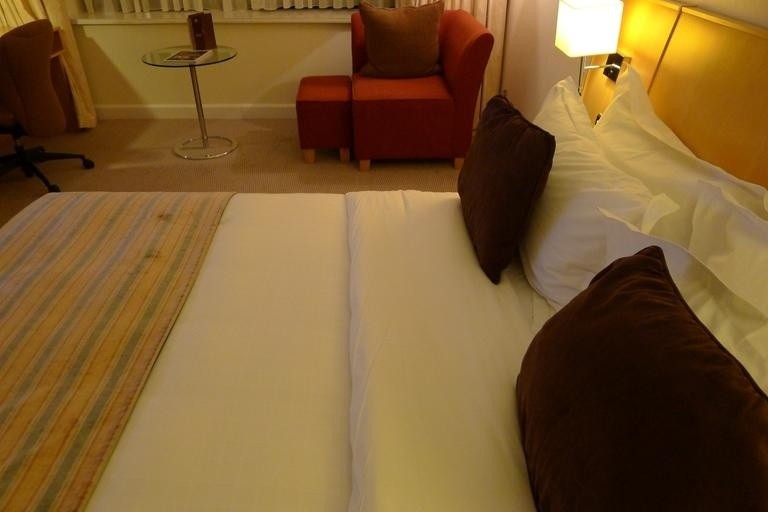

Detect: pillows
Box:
457 90 556 287
513 246 764 512
518 69 768 390
354 1 445 79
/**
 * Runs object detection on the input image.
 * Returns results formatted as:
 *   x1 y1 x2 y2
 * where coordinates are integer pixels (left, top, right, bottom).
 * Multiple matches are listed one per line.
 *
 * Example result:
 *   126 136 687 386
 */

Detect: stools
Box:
295 74 353 166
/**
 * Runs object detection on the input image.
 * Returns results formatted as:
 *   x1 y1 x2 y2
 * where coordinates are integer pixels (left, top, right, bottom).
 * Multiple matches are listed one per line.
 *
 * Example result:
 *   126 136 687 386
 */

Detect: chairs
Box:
347 5 495 173
1 17 95 192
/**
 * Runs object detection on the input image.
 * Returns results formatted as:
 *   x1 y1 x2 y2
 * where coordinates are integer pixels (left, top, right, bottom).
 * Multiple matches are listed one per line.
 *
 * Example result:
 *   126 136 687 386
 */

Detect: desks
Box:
49 25 80 136
141 43 239 161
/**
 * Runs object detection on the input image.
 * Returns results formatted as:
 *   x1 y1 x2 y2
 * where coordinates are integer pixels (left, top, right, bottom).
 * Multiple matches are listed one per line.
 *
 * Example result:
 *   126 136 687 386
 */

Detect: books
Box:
163 47 212 63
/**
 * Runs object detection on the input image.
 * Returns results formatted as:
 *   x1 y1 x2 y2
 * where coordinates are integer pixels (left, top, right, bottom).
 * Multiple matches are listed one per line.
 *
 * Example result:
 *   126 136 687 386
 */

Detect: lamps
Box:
555 0 632 96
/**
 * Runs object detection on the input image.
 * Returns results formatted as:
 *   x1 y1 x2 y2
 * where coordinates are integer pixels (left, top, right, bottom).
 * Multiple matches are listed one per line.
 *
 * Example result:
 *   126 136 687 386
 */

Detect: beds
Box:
2 1 768 512
